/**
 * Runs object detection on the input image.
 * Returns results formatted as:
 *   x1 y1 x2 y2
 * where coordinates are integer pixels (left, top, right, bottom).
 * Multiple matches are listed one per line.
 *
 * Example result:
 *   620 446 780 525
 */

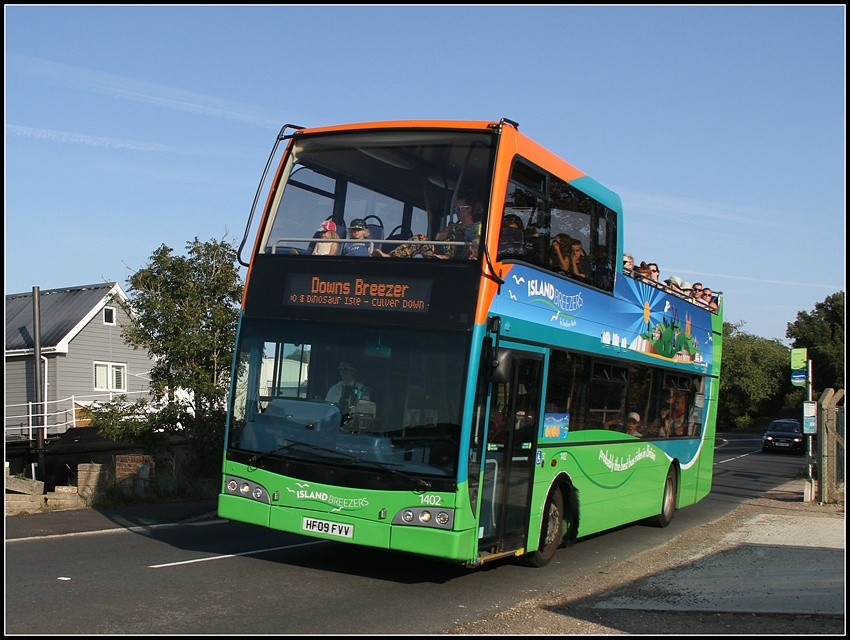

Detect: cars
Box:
762 419 805 454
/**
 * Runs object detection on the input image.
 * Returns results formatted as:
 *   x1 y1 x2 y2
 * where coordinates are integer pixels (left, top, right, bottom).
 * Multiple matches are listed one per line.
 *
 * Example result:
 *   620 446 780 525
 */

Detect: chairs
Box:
309 214 588 281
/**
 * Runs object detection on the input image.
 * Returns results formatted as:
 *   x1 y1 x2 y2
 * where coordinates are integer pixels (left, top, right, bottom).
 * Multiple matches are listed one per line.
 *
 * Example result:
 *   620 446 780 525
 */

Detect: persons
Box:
288 194 594 279
622 255 718 315
603 412 643 437
324 358 371 431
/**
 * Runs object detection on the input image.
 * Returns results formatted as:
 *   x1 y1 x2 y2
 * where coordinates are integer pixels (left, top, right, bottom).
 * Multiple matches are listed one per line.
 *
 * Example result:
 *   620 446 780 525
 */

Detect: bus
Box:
216 116 726 569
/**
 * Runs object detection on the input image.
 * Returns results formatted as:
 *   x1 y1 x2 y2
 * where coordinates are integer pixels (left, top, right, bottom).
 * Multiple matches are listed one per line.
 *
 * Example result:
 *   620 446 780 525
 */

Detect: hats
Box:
628 412 640 422
664 275 681 288
348 219 366 229
315 221 336 231
680 282 693 289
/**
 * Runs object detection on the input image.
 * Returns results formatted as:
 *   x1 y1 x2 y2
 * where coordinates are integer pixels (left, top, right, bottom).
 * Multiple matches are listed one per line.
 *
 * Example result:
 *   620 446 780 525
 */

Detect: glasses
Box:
694 288 702 292
337 367 348 371
675 400 680 404
455 206 470 212
704 294 711 296
651 269 656 273
623 261 628 265
